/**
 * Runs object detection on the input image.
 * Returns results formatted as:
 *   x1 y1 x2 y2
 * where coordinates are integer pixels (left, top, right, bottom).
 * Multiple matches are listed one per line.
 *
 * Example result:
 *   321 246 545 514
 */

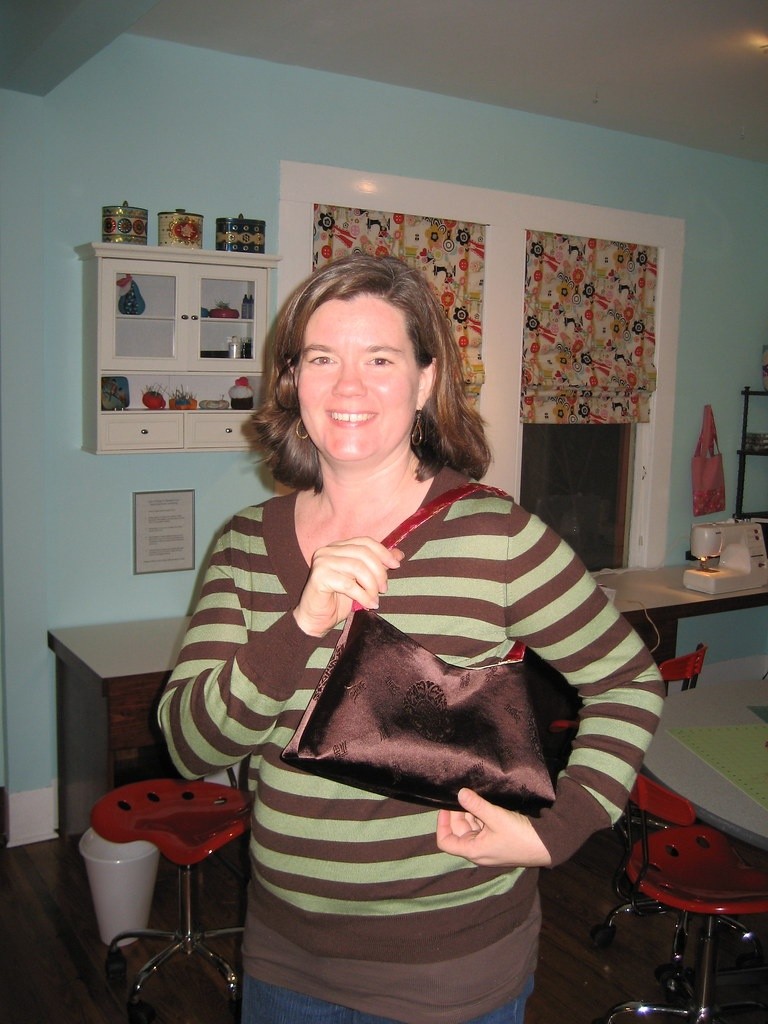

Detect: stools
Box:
88 778 256 1024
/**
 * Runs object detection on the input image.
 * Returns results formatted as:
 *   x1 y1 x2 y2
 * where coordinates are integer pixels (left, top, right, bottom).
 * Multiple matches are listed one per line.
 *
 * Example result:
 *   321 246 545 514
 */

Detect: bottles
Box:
241 336 252 359
229 377 255 410
242 294 254 318
229 336 241 358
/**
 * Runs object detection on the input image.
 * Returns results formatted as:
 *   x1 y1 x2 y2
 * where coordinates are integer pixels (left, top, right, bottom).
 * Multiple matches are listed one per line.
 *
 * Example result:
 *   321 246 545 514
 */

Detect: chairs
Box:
551 643 768 1024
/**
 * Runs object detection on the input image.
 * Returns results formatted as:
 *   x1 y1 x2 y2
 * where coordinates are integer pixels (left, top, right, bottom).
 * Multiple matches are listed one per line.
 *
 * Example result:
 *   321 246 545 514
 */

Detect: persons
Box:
158 255 663 1024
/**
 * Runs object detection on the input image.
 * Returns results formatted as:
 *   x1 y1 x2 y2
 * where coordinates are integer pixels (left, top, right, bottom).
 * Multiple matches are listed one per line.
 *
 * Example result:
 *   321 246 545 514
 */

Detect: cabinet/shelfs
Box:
74 242 281 455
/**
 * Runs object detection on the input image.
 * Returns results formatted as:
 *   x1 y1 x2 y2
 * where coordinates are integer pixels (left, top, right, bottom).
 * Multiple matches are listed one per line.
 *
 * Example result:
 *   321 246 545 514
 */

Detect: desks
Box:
45 563 768 849
643 678 767 851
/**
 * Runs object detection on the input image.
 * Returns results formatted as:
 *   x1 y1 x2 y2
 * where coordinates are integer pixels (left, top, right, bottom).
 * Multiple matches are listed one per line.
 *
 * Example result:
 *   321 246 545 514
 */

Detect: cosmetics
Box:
230 335 252 359
199 293 254 319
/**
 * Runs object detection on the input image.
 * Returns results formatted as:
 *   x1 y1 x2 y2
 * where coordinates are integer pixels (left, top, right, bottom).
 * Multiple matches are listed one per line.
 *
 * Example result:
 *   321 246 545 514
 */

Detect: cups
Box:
79 827 159 948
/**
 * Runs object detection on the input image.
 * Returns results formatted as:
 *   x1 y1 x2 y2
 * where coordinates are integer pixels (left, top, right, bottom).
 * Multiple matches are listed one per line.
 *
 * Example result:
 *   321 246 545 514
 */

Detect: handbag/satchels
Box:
281 482 556 818
692 403 725 516
